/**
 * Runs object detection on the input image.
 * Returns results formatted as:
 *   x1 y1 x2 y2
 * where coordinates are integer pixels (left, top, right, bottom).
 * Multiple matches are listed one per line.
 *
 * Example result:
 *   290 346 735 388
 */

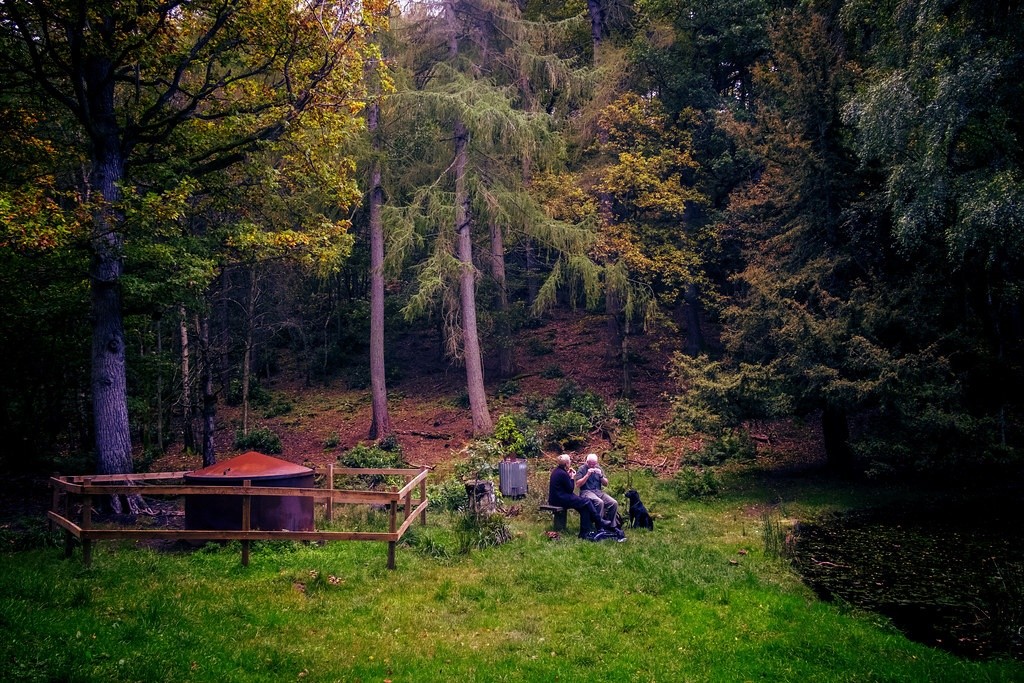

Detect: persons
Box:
575 454 619 533
548 453 612 540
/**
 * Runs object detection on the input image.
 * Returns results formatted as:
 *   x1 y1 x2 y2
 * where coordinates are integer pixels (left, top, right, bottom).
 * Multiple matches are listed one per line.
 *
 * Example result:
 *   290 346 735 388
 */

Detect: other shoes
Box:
579 532 594 540
596 518 611 527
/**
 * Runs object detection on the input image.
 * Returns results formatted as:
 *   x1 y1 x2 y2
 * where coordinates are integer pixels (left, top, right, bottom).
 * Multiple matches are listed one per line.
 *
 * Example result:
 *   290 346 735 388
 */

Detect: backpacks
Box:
602 509 622 530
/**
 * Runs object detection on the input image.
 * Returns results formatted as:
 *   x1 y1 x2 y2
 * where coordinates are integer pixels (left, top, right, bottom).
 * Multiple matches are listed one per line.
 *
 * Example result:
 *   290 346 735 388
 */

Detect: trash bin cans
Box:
498 459 528 496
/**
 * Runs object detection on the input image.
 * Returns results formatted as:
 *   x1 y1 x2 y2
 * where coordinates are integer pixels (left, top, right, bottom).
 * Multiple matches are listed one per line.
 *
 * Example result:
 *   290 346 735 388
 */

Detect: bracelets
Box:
599 475 603 478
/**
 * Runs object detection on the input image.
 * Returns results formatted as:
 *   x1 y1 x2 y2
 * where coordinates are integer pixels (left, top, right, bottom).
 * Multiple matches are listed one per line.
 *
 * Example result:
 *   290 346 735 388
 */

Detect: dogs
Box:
625 490 653 532
